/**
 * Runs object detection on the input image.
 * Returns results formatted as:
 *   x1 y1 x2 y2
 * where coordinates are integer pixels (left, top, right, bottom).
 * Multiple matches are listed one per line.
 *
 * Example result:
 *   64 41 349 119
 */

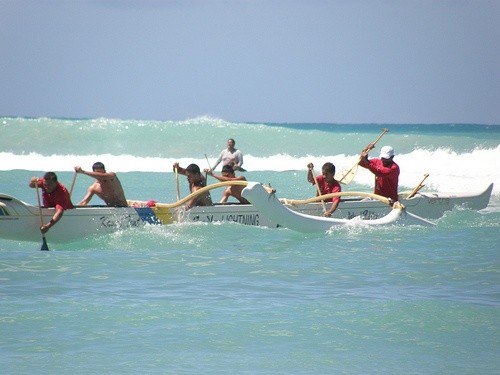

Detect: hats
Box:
380 146 395 159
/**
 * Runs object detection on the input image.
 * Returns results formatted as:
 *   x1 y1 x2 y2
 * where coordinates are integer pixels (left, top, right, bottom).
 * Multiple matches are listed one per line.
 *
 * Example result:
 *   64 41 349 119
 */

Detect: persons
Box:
357 143 400 203
306 162 341 216
28 172 73 233
210 138 243 171
173 163 212 213
204 165 250 205
74 162 128 207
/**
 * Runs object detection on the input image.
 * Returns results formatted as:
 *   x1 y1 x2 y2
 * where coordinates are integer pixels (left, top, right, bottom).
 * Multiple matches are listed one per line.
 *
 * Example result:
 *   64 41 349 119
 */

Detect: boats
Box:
0 181 495 245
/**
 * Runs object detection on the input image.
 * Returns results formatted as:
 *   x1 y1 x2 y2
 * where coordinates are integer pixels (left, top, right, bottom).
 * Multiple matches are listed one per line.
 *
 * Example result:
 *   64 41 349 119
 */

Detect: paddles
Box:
205 169 207 186
309 166 328 217
338 128 390 185
175 164 181 222
406 174 429 199
69 171 78 198
34 182 49 250
